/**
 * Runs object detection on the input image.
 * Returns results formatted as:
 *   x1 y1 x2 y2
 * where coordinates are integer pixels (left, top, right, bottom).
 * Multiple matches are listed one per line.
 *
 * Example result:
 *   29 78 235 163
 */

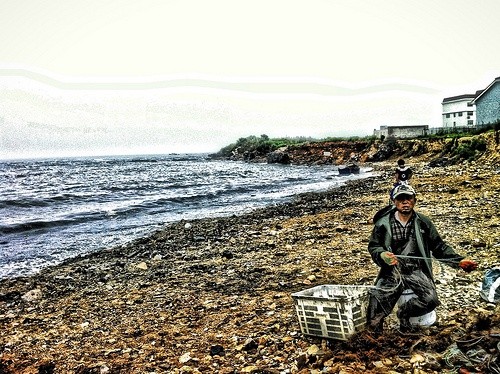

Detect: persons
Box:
366 184 478 331
395 159 413 184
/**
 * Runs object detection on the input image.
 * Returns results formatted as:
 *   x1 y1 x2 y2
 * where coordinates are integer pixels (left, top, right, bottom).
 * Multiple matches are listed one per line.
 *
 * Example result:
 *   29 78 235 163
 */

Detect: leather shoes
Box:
396 309 411 329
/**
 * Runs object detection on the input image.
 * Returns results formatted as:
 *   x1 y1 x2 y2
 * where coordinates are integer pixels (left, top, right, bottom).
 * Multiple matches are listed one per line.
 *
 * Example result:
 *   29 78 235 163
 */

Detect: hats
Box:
392 184 416 199
